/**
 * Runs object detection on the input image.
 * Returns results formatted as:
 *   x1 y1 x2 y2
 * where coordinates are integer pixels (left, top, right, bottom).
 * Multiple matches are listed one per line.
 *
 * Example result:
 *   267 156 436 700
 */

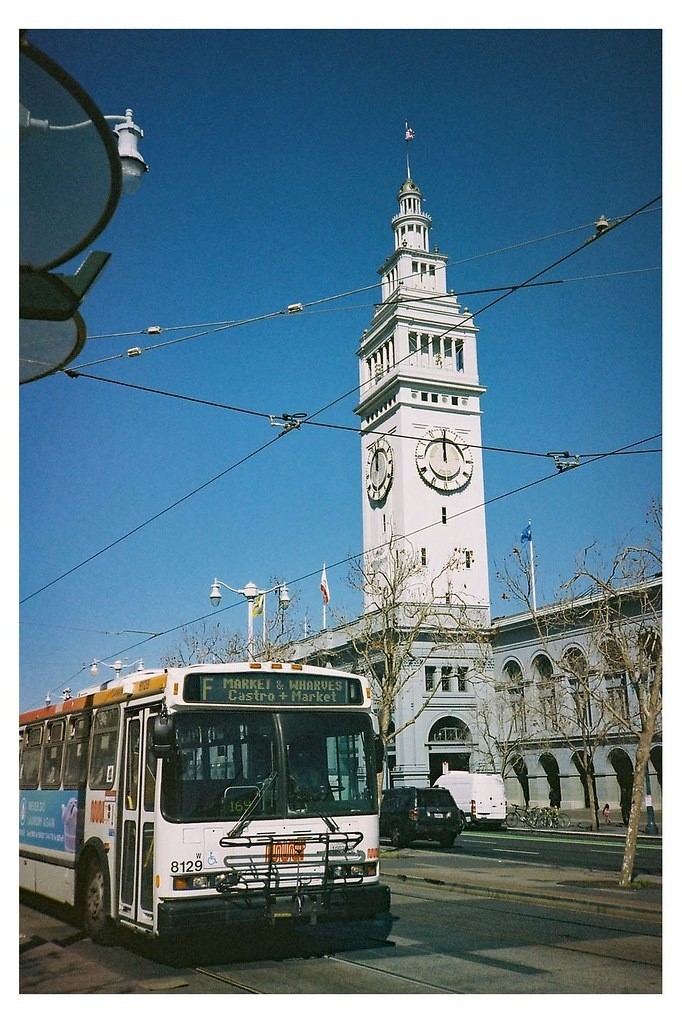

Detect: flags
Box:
520 523 532 543
320 562 330 603
252 594 264 616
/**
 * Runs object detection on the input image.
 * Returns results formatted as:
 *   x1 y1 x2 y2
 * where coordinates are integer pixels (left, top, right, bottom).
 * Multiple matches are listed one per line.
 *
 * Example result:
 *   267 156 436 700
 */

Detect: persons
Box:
619 786 632 826
603 804 611 823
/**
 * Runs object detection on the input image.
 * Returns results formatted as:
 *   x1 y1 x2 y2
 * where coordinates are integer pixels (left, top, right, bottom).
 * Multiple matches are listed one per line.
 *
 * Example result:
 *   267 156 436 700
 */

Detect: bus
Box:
20 657 392 947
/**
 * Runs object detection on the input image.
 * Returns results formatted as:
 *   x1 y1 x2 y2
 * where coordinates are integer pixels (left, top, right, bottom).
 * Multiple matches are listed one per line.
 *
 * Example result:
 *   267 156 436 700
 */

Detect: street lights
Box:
90 658 145 678
210 578 291 662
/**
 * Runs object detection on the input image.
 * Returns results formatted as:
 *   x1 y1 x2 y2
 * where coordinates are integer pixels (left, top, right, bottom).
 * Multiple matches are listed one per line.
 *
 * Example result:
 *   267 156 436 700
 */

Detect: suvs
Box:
379 784 462 849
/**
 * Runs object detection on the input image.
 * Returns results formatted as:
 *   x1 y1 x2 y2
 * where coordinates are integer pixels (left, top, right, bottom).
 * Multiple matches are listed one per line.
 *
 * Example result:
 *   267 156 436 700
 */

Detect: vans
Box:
432 773 507 831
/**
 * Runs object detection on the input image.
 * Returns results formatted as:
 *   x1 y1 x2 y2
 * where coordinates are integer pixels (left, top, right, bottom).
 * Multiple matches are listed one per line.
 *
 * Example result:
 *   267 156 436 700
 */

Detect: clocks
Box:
366 439 393 501
415 428 472 490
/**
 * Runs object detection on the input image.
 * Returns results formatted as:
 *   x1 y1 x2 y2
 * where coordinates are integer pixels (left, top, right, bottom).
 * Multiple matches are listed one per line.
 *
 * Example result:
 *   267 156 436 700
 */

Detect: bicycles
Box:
506 803 571 830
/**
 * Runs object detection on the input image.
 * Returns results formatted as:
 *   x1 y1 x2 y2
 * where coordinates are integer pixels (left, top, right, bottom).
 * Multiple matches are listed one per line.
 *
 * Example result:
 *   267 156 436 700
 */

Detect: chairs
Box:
178 778 265 818
